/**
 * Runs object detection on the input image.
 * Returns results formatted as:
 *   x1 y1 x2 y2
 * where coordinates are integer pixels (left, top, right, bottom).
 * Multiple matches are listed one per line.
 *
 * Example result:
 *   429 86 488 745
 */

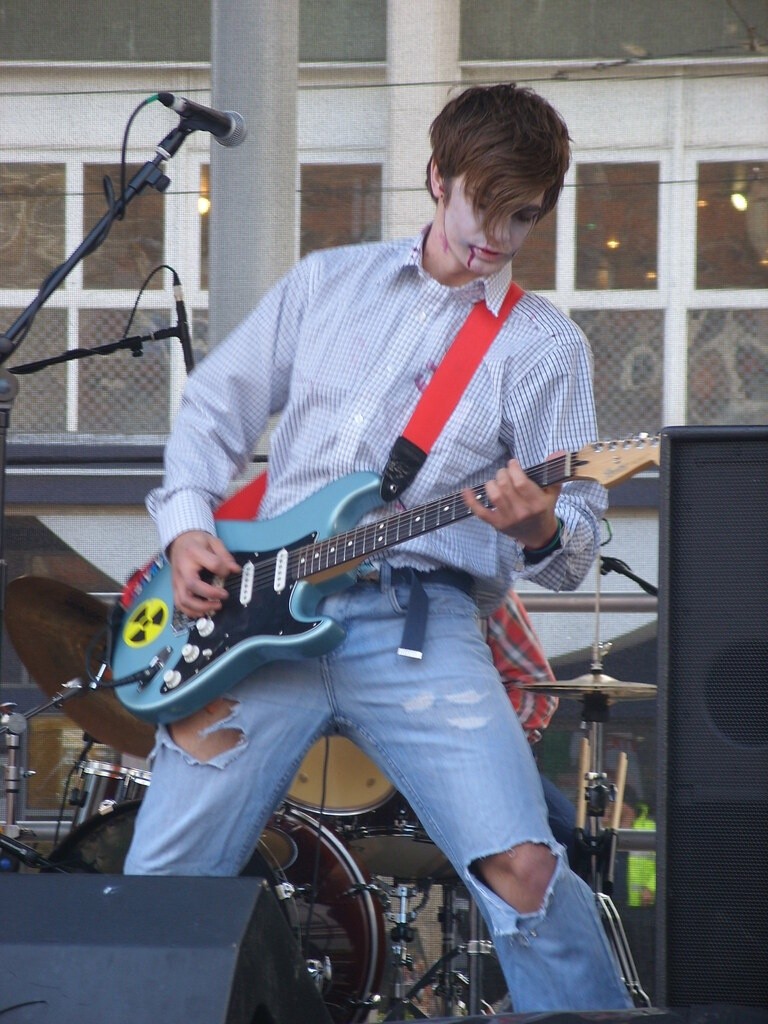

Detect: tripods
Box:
374 873 500 1024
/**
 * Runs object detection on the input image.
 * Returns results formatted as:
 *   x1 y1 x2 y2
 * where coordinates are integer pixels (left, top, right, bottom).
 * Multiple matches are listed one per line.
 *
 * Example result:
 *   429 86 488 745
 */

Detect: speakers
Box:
1 874 338 1024
655 422 767 1018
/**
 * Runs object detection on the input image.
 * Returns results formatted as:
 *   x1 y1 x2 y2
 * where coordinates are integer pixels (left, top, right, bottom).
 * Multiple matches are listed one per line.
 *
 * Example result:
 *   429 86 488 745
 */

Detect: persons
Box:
120 85 633 1012
484 587 589 845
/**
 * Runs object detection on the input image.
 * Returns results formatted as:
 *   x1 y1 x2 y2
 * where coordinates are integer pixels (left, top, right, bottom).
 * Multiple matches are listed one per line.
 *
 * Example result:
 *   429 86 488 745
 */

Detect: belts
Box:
347 562 475 660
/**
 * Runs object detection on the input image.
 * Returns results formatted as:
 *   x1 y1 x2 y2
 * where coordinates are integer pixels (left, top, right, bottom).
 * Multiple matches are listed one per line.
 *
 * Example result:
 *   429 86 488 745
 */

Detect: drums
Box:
36 795 386 1024
68 758 153 832
282 732 398 819
331 792 452 882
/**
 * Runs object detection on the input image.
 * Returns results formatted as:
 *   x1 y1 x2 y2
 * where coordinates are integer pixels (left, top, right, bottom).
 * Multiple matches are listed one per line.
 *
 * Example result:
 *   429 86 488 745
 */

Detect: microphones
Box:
172 274 194 374
103 668 152 688
156 93 247 146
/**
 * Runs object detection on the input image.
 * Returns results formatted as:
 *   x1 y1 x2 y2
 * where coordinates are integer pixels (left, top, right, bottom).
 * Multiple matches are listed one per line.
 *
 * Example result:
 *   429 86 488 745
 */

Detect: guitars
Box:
111 429 661 728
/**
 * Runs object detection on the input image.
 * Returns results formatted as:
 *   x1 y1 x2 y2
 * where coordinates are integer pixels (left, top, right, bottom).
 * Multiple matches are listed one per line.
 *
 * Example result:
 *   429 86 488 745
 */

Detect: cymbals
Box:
518 672 657 706
2 572 158 761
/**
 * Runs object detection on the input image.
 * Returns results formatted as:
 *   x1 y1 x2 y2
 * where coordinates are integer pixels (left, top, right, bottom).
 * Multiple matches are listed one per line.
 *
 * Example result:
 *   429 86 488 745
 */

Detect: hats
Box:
623 784 638 808
